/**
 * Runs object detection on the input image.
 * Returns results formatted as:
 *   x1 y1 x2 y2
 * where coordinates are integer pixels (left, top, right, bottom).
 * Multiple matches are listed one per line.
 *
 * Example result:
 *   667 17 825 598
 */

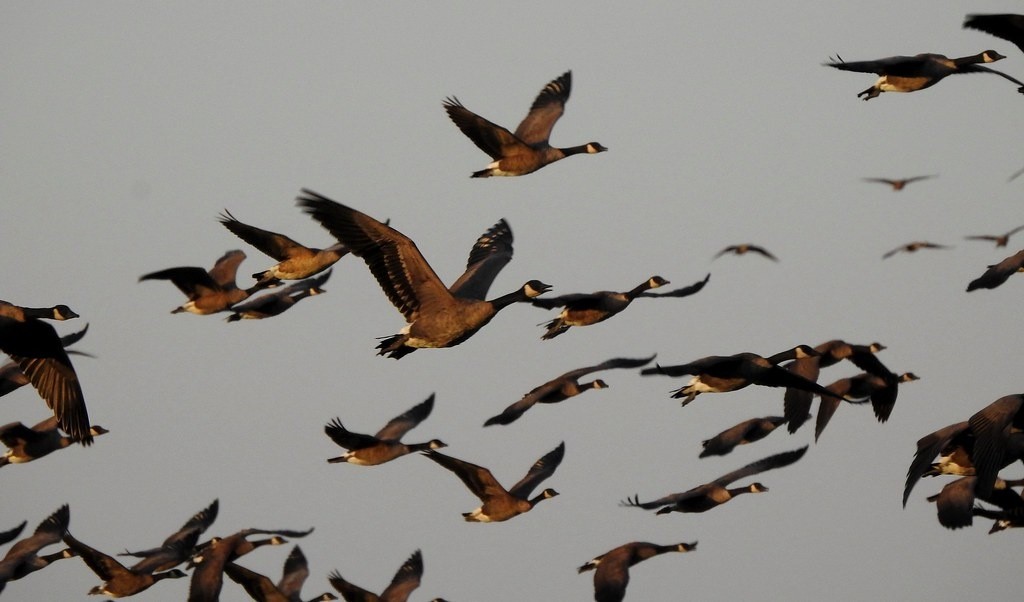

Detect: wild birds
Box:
966 249 1024 293
0 497 448 601
882 242 953 259
825 12 1024 100
963 225 1024 247
441 70 608 178
902 393 1024 534
138 186 923 523
577 540 698 602
0 296 110 469
859 175 940 192
